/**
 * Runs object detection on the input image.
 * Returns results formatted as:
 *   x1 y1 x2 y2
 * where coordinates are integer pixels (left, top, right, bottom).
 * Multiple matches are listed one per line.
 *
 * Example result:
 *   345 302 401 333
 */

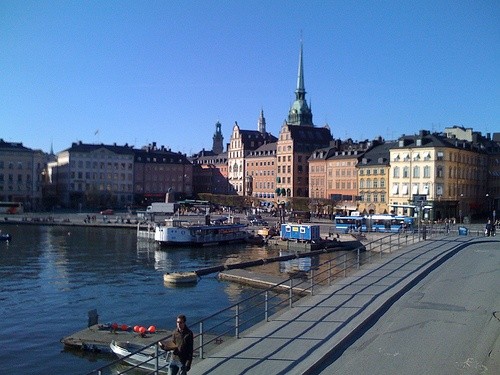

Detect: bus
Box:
174 199 212 216
334 213 416 233
0 201 24 214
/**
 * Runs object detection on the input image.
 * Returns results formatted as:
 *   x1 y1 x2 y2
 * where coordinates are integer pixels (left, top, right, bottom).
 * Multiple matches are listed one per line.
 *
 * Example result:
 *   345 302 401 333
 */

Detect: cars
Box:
100 209 114 215
245 215 261 220
209 216 229 225
250 219 268 226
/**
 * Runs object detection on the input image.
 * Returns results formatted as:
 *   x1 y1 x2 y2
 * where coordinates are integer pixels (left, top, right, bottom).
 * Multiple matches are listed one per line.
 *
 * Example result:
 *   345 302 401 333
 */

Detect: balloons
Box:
112 324 155 333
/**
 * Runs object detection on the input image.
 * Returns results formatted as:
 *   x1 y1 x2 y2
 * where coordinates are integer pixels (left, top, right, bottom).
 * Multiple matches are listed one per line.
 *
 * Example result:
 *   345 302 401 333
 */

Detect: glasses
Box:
177 321 184 323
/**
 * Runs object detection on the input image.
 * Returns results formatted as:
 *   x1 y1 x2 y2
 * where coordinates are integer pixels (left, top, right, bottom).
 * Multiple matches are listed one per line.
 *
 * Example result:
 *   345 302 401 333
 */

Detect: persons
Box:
168 314 193 375
486 220 492 237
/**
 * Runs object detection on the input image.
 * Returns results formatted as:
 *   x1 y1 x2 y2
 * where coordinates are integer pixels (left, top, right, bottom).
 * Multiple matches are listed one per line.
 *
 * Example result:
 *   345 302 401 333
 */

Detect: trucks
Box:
147 202 175 214
288 210 310 222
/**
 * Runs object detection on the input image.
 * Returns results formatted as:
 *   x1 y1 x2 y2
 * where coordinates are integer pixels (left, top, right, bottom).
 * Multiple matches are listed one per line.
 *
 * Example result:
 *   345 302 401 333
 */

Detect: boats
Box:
152 218 253 247
110 339 176 375
